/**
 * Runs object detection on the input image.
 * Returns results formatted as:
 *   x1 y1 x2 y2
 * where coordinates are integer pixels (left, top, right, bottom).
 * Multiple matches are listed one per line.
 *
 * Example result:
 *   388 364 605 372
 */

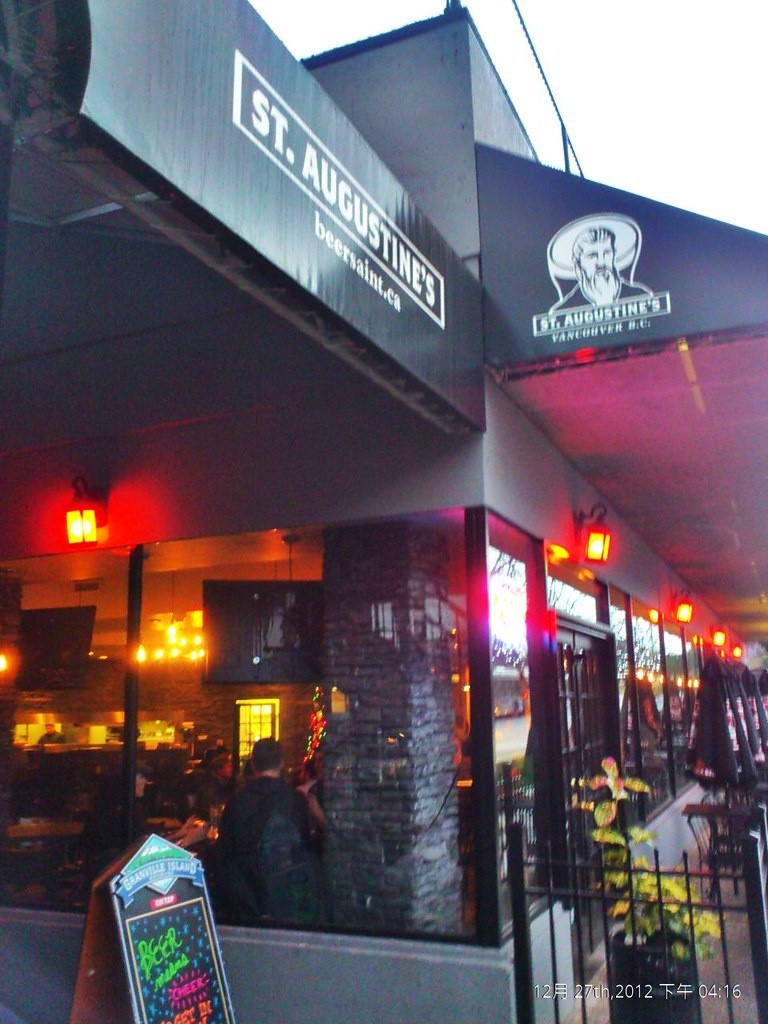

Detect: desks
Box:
681 804 742 818
6 823 81 904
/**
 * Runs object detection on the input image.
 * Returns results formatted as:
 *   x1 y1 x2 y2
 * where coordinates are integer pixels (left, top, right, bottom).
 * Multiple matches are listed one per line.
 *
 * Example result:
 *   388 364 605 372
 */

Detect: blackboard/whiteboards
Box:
105 858 236 1024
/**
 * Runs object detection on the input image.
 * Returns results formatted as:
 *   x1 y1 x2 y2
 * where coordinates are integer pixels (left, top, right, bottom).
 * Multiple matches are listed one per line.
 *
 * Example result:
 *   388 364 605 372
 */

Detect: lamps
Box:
672 589 695 624
577 502 612 565
65 476 108 547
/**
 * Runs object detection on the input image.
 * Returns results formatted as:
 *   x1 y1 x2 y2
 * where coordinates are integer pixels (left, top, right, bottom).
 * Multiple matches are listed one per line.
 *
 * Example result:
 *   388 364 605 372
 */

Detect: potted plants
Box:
579 755 727 1024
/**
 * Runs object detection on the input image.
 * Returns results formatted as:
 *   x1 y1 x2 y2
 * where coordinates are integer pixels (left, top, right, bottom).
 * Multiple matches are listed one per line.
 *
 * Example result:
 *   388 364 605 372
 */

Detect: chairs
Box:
687 811 741 899
252 834 318 922
701 789 749 870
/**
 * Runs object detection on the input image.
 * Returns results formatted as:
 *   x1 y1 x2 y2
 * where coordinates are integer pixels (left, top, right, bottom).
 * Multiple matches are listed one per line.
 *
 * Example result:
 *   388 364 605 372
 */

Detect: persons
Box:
118 723 146 751
37 723 66 744
0 737 327 907
218 737 311 925
